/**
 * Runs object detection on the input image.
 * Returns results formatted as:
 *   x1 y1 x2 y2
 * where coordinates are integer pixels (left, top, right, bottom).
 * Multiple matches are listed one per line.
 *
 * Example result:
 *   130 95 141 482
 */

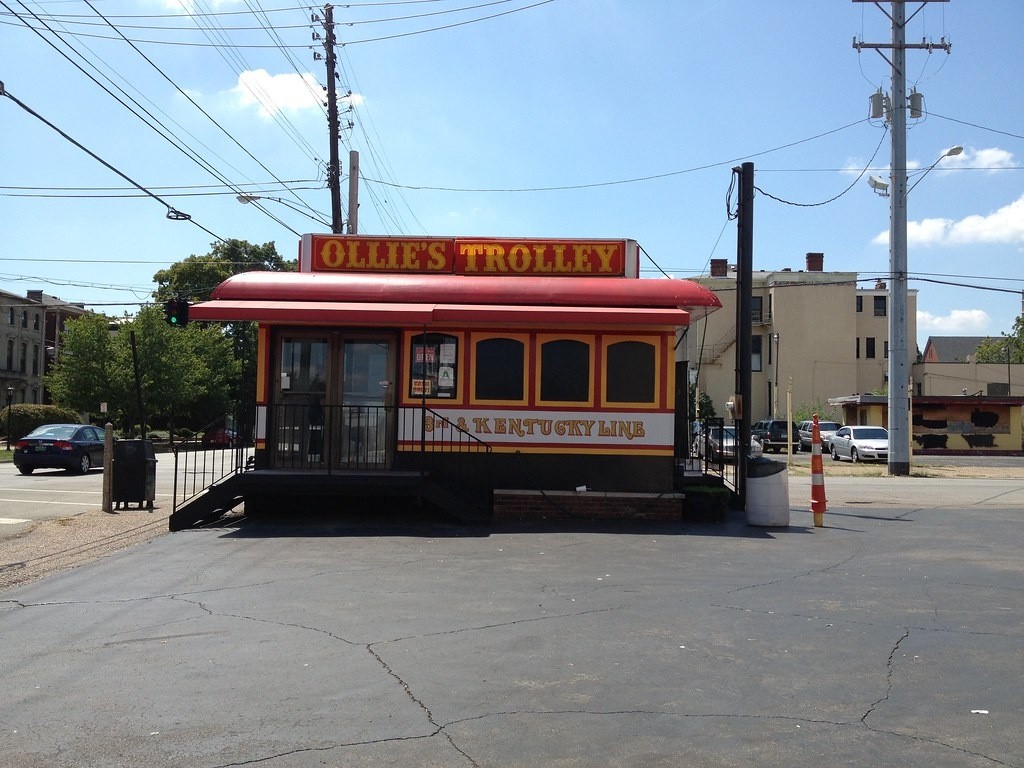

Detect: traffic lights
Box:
162 299 188 327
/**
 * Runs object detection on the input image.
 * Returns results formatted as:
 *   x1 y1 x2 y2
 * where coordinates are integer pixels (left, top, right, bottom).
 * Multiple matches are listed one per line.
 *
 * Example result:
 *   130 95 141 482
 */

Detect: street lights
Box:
887 145 963 476
6 385 15 451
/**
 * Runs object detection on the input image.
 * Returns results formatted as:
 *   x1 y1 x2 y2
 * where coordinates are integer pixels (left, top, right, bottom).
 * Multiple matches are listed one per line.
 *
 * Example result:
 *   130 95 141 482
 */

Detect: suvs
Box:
751 418 802 454
796 419 843 451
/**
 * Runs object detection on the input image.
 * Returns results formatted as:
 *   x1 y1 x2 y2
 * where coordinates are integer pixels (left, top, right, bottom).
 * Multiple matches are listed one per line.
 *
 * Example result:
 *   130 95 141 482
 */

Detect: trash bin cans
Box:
745 459 790 527
112 438 158 509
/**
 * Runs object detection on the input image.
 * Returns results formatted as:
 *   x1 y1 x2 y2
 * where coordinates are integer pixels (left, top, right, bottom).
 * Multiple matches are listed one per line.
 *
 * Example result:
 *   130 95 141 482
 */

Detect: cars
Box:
13 423 118 475
828 425 889 465
693 426 763 462
201 426 242 449
